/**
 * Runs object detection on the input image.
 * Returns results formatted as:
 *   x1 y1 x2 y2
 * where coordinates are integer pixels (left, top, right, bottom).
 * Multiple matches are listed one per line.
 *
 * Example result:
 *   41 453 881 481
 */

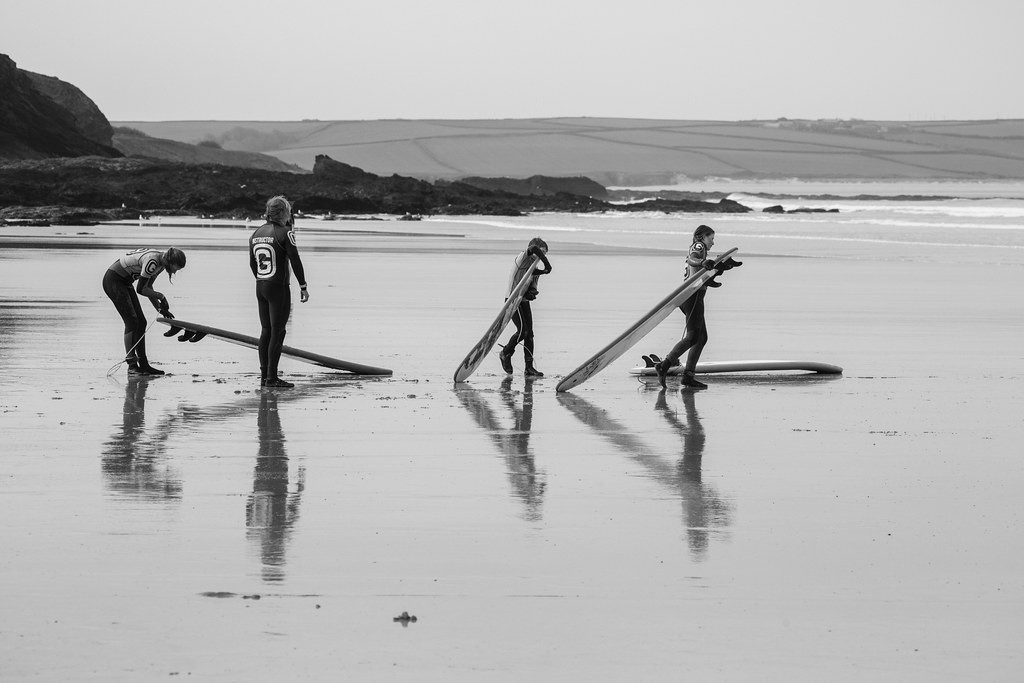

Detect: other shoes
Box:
499 350 514 374
524 367 544 377
260 379 267 386
127 363 141 374
681 374 708 388
265 377 294 388
139 362 165 376
655 362 668 388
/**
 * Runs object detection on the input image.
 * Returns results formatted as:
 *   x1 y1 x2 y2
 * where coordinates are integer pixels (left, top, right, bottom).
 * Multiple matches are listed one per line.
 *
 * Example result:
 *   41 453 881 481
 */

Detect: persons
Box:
655 225 724 388
250 195 310 387
499 238 552 376
101 248 186 375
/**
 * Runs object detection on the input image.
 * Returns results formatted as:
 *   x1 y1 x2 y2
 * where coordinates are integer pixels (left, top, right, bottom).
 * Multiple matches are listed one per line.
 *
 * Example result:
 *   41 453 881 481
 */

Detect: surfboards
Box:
156 317 393 375
555 247 744 392
453 257 540 382
628 354 844 375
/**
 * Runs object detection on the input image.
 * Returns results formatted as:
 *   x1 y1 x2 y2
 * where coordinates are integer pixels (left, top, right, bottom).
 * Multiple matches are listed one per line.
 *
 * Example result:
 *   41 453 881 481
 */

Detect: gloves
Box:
702 259 715 270
158 306 175 319
160 296 169 310
524 244 535 255
